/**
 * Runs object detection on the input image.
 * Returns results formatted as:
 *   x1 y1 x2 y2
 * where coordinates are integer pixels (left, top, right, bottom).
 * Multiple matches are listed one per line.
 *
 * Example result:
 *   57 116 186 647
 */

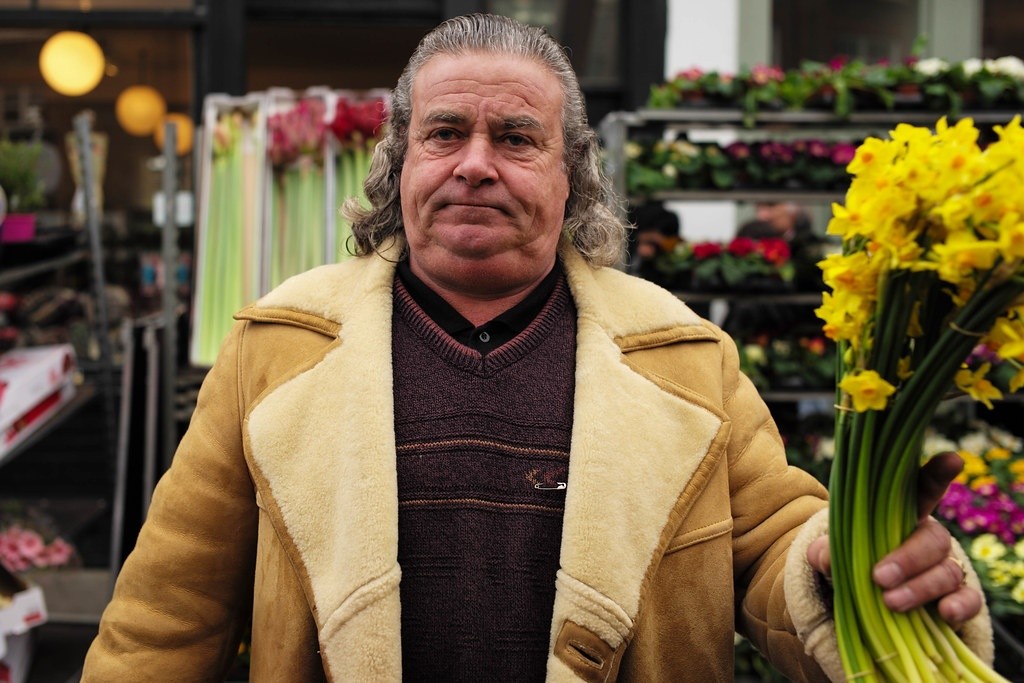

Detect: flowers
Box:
265 57 1024 619
0 524 77 578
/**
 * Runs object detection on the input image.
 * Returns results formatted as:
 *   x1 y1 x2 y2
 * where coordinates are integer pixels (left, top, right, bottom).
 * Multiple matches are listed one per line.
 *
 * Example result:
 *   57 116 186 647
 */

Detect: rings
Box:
947 556 967 585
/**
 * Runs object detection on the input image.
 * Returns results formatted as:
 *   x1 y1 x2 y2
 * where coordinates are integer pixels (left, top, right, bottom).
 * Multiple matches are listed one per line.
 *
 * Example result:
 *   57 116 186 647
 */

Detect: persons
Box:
81 6 994 683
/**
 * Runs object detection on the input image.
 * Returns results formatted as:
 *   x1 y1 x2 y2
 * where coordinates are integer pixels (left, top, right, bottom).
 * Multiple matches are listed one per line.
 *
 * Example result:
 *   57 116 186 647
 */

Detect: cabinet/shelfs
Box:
607 103 1024 396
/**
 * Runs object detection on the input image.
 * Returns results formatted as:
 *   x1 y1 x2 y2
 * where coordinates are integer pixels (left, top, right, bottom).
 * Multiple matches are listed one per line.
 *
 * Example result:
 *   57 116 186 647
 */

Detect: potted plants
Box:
0 136 47 242
